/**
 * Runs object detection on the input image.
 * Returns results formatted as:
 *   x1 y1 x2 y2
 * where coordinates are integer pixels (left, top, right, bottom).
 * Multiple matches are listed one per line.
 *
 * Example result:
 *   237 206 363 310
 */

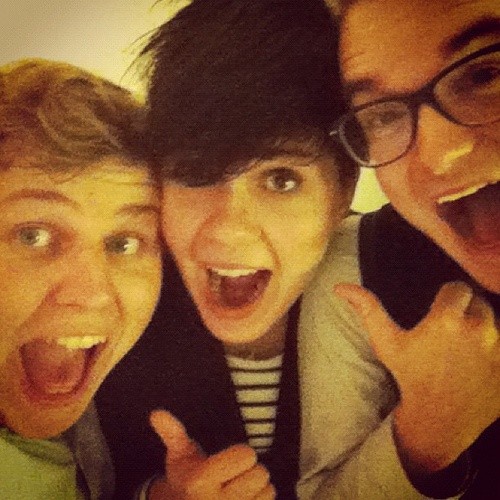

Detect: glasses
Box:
328 43 500 168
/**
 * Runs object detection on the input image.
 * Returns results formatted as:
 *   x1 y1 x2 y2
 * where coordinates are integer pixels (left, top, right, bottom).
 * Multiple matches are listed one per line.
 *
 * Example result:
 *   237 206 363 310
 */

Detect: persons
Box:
2 57 163 500
64 0 369 500
296 0 499 500
